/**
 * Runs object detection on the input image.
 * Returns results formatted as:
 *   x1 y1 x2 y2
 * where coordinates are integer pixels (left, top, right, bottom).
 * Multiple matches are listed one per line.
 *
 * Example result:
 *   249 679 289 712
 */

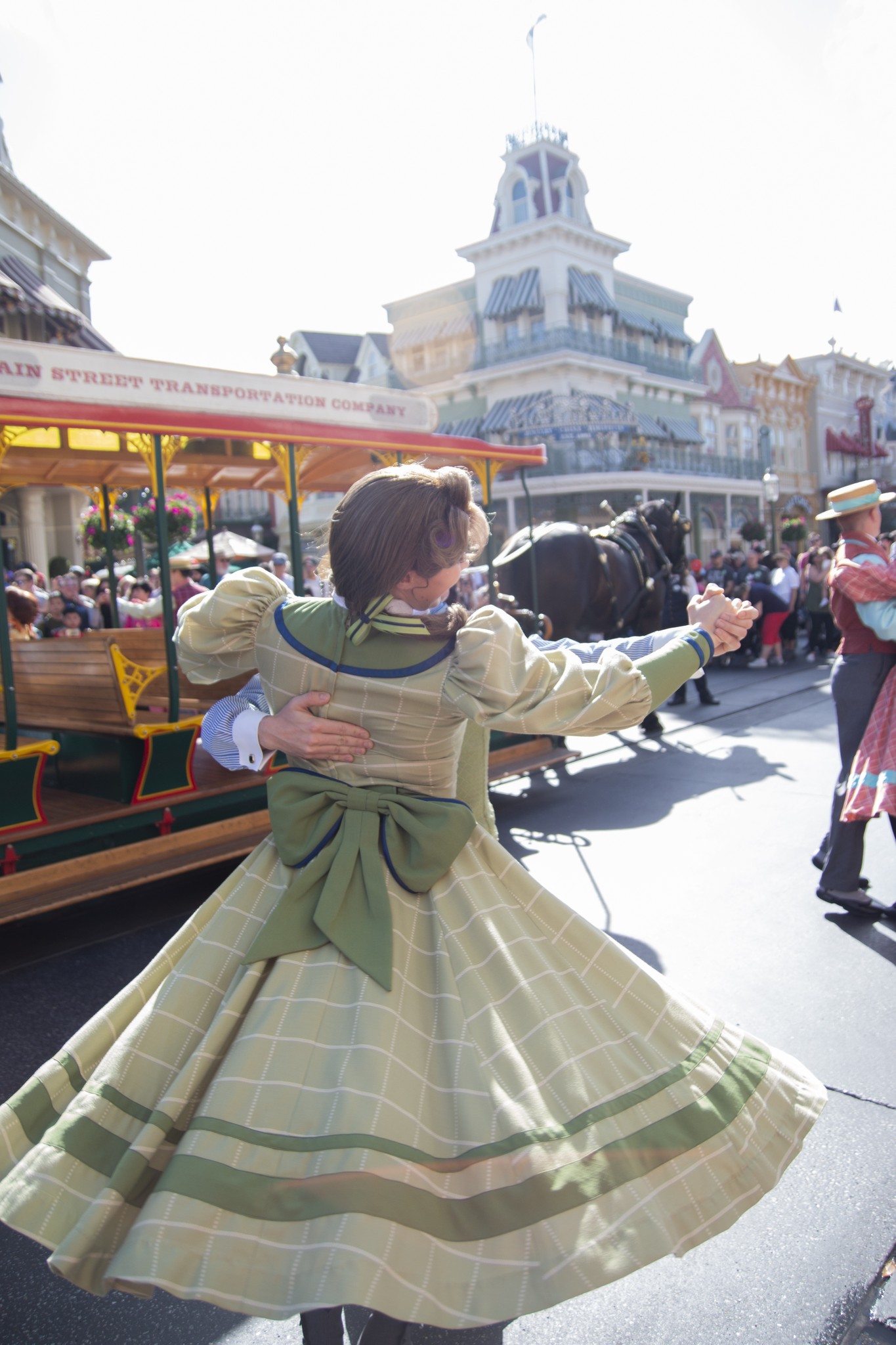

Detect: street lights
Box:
763 466 781 556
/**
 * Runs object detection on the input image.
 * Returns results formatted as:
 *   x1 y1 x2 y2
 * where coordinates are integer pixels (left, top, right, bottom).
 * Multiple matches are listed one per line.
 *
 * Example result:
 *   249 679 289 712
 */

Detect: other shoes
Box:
644 721 662 734
813 849 869 888
704 696 720 705
668 697 685 705
747 657 768 668
817 885 886 918
806 653 815 662
776 658 784 665
824 651 836 660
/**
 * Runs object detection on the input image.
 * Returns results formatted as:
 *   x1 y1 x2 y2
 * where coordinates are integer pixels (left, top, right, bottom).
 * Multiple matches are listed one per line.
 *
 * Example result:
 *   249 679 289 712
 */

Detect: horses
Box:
486 487 724 736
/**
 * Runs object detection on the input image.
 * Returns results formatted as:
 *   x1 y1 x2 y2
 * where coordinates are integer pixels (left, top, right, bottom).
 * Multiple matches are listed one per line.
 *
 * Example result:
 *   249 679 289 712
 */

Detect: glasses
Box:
15 578 30 585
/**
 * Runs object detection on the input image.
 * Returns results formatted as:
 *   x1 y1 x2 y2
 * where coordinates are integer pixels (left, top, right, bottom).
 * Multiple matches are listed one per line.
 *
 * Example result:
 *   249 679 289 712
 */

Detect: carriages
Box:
1 338 691 921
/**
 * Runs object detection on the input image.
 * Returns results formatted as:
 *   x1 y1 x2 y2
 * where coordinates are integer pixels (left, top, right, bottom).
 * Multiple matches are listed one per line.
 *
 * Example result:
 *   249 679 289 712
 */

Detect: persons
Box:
812 479 896 922
4 550 230 641
0 462 829 1345
669 533 836 704
270 552 331 597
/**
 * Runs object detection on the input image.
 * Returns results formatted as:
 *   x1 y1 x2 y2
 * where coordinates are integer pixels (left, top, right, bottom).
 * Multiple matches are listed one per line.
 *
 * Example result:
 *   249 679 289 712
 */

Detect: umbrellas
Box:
170 525 275 565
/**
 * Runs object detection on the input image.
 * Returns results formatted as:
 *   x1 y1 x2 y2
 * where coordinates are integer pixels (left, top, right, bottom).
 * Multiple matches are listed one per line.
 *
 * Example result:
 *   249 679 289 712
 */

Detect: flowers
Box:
134 490 208 547
75 492 139 556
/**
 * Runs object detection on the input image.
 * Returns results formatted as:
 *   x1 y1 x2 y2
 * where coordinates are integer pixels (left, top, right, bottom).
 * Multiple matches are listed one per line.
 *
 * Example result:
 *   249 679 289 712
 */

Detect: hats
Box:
815 479 896 521
82 577 101 588
70 565 85 575
272 553 288 565
169 555 200 570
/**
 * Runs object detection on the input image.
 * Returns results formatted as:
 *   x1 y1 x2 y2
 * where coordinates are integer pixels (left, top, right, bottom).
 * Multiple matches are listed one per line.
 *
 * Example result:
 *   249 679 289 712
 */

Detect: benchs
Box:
0 626 289 833
